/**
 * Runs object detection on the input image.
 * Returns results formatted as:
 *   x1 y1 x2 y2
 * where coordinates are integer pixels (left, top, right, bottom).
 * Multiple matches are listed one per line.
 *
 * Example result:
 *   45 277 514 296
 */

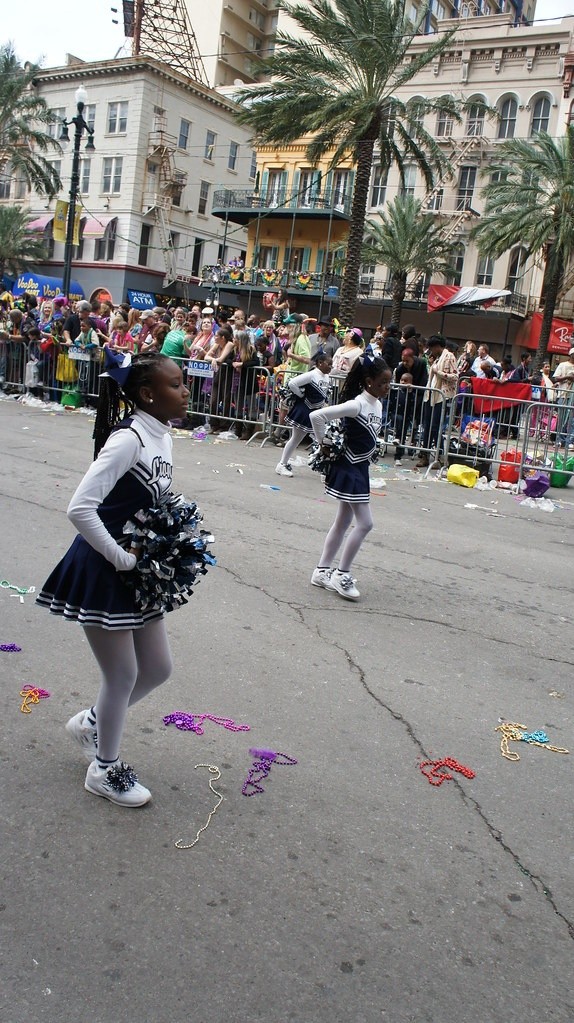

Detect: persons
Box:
228 256 244 267
32 352 191 807
308 354 392 600
263 289 290 320
273 351 333 477
0 293 574 467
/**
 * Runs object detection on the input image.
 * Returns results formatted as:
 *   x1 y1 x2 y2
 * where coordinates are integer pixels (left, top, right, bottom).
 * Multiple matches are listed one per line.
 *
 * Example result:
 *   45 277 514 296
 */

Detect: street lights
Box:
59 82 95 306
206 274 218 320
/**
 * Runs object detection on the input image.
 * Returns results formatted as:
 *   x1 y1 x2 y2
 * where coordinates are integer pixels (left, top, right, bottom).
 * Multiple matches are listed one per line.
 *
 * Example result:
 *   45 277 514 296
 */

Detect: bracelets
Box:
270 301 272 303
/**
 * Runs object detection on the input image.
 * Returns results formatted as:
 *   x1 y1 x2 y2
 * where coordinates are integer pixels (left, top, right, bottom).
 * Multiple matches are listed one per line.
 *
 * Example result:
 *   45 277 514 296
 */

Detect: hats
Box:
53 298 64 307
569 347 574 355
403 324 416 336
350 328 363 336
201 307 213 314
227 315 235 321
283 313 303 324
119 310 128 321
139 309 153 320
153 307 165 313
317 315 335 326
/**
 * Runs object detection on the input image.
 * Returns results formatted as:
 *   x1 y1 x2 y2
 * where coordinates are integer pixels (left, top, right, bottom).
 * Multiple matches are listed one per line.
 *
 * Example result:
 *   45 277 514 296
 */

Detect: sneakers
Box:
311 568 337 591
328 568 360 599
84 761 151 807
66 710 98 763
275 462 294 477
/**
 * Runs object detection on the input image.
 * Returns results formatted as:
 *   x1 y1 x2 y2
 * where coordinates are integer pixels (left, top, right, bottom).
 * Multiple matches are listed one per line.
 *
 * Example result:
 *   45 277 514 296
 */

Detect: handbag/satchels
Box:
550 452 574 487
522 455 550 479
498 445 522 483
56 348 78 382
442 438 492 482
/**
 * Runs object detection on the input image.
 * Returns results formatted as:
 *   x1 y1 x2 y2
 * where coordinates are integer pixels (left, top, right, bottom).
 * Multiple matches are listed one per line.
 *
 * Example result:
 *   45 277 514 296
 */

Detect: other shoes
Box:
568 444 574 450
173 400 441 468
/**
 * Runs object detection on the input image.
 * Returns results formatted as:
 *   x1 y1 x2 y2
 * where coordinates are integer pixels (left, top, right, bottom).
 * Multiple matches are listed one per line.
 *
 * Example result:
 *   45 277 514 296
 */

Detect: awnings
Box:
66 216 86 240
82 216 118 240
23 215 55 239
